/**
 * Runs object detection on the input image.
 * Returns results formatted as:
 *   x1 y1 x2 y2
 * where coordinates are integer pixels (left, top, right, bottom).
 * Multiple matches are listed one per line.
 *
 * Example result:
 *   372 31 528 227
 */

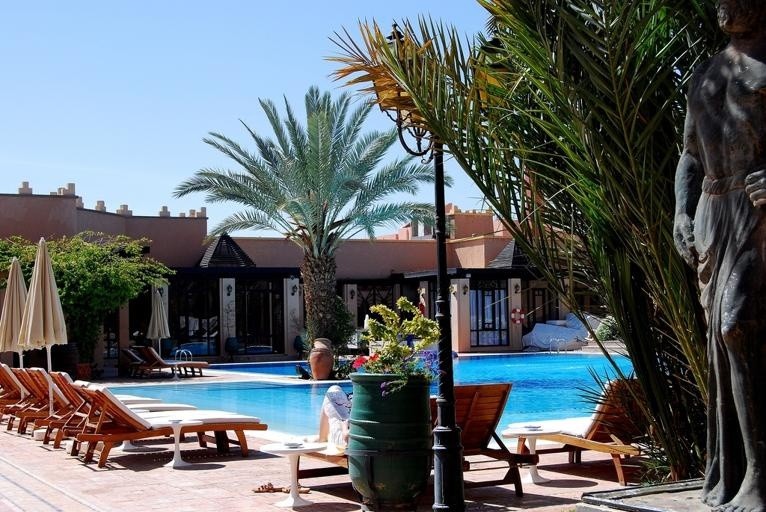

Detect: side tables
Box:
260 443 328 509
503 422 561 484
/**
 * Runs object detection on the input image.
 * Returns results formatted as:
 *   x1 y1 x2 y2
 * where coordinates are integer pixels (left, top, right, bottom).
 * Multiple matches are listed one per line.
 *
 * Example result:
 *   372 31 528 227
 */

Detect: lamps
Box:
463 284 468 295
226 284 232 296
292 285 297 295
350 290 355 299
158 287 163 296
515 283 519 293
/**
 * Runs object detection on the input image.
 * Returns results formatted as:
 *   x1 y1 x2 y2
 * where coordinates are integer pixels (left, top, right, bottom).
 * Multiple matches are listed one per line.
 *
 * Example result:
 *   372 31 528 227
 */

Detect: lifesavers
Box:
510 309 524 324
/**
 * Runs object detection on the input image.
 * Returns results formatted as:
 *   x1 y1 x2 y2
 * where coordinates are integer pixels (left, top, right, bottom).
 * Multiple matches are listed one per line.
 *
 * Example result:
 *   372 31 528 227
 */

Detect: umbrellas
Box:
14 235 68 418
0 256 29 402
143 288 171 360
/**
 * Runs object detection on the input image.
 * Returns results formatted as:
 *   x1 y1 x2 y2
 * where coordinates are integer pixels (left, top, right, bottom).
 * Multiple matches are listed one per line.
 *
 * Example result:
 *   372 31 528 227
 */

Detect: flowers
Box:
344 297 458 396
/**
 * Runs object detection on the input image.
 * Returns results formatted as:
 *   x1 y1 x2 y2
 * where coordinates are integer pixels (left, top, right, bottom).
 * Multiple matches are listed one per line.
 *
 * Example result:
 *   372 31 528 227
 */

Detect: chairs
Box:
503 378 649 487
123 345 208 380
0 363 267 467
298 383 539 497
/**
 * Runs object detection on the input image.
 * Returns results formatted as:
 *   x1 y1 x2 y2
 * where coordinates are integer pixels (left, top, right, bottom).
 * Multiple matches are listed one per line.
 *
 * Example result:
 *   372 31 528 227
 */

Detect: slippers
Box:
252 482 285 493
281 482 311 493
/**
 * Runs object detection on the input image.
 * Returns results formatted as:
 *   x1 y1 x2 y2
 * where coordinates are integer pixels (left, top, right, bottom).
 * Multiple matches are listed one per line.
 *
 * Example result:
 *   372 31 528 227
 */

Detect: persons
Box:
668 0 766 512
301 384 353 449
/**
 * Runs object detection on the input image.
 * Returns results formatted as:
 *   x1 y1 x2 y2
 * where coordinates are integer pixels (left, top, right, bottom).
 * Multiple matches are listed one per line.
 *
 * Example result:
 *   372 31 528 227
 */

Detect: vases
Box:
345 374 431 512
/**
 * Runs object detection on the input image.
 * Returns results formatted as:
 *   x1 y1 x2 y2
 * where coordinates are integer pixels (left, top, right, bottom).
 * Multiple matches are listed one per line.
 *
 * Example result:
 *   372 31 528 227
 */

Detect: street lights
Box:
372 23 465 512
